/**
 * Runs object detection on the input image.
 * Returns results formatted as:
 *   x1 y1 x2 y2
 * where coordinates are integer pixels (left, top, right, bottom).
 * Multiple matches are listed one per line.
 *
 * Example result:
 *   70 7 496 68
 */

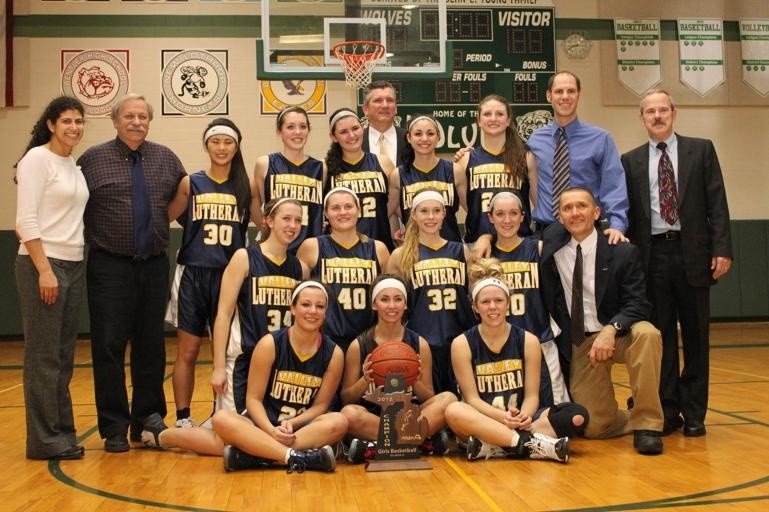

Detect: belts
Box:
112 252 166 263
653 231 680 240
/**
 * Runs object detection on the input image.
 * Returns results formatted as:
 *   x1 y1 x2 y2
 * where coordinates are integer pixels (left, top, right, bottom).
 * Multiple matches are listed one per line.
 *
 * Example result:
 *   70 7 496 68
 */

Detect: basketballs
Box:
370 341 418 387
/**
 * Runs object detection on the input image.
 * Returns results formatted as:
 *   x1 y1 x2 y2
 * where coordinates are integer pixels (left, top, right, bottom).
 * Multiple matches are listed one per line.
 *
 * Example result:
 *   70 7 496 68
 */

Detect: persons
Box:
10 95 91 457
75 94 189 452
140 60 733 473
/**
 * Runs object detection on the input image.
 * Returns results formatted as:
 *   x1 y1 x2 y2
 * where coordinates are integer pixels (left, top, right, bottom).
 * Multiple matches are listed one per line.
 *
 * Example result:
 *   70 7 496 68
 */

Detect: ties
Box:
130 150 152 257
378 135 395 178
571 245 585 348
656 142 679 225
552 126 571 223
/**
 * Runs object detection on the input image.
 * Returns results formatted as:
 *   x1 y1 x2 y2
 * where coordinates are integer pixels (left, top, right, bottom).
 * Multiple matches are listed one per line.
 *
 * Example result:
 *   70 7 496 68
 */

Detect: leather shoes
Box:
684 419 706 437
29 445 85 460
660 413 684 436
103 434 130 452
633 429 663 455
129 433 142 441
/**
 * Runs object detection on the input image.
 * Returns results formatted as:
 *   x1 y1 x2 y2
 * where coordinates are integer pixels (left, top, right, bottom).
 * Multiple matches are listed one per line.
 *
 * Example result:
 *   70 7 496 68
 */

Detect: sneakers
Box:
176 417 196 430
223 445 274 471
467 435 516 460
422 432 450 456
287 445 335 473
348 438 378 465
518 433 570 463
139 412 168 450
